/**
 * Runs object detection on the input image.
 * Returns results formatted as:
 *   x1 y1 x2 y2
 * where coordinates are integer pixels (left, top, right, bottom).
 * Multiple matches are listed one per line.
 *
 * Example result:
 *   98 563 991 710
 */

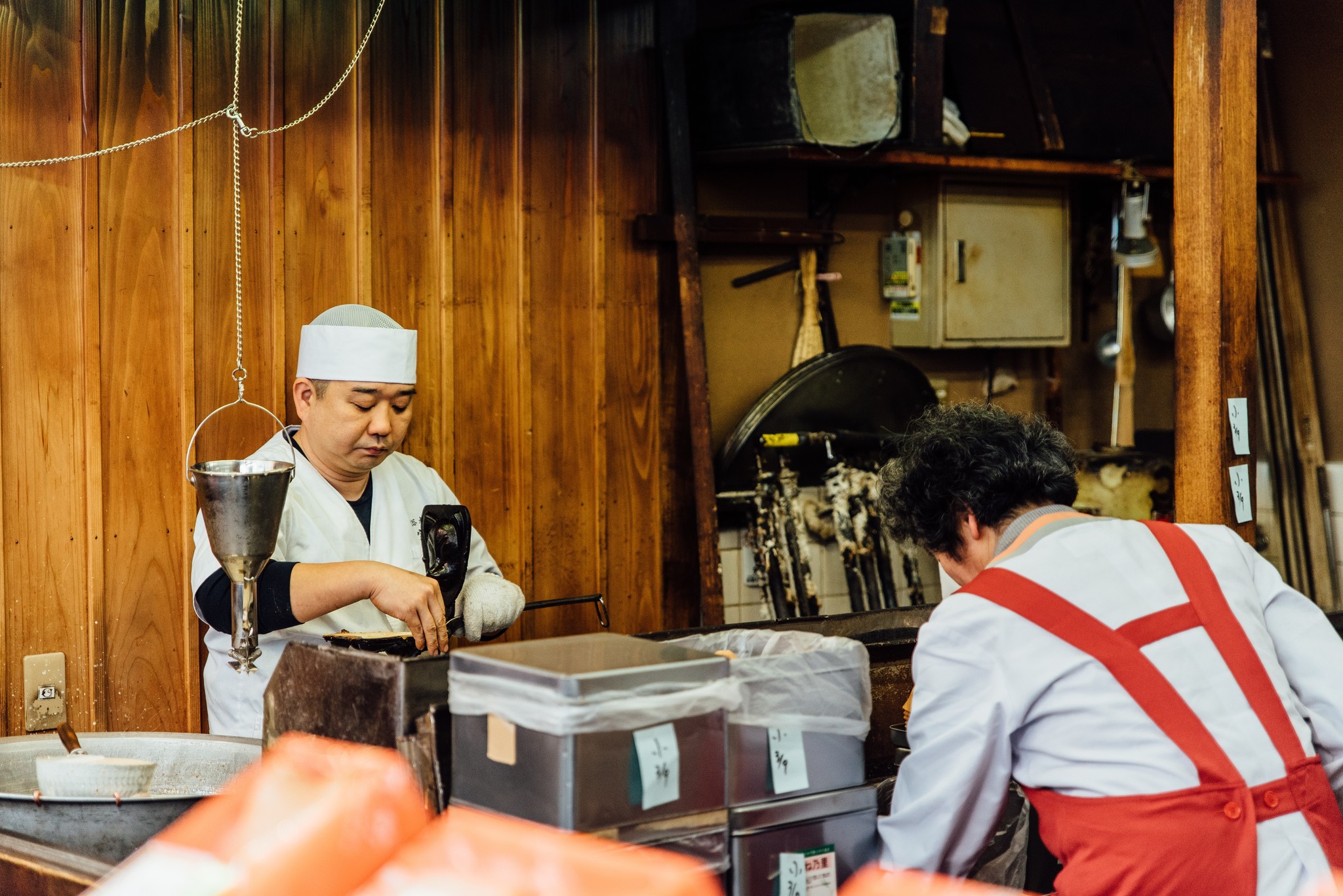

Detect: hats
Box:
296 304 416 385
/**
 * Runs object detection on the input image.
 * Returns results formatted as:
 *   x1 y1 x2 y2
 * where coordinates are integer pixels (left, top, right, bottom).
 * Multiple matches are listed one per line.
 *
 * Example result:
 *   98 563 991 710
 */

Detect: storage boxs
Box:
724 784 877 896
447 633 731 837
586 806 733 896
658 628 874 811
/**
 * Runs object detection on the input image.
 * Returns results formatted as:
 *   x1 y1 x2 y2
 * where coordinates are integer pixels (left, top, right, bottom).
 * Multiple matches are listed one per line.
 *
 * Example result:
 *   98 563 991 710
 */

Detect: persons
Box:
866 394 1343 895
189 302 527 741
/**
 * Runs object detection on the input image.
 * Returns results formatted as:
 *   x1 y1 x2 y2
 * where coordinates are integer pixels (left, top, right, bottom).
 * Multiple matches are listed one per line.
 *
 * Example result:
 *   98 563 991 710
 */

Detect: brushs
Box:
790 250 826 368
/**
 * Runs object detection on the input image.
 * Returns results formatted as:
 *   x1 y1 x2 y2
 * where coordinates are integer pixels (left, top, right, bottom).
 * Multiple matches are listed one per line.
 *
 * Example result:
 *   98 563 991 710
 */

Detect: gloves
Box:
451 572 525 642
